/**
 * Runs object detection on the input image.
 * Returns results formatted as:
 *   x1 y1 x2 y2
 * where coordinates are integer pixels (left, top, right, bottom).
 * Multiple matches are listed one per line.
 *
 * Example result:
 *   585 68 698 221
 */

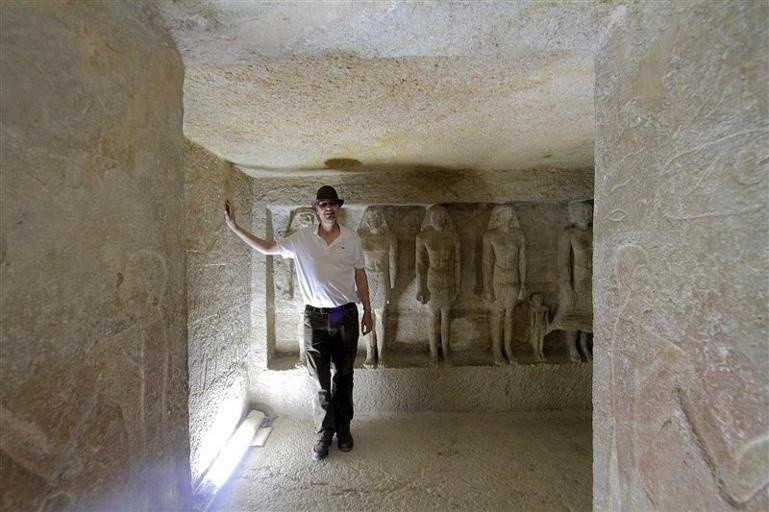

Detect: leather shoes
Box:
336 427 354 452
311 431 334 461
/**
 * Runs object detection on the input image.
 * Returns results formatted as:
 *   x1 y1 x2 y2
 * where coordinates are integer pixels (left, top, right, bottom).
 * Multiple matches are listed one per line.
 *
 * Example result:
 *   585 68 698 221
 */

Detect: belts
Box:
306 302 354 314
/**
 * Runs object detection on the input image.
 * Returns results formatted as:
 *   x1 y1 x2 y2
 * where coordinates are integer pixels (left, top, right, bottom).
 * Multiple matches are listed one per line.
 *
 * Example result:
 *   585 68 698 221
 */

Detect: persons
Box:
553 199 595 363
414 207 462 361
355 206 400 369
524 288 552 362
221 181 376 462
478 202 533 366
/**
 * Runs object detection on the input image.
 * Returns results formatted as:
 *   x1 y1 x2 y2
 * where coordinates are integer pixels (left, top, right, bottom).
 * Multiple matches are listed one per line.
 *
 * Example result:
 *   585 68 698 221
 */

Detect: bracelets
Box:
362 305 372 311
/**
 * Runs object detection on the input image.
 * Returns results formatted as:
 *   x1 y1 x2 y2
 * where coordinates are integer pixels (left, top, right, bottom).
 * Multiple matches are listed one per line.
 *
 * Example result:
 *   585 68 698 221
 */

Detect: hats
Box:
311 185 344 209
